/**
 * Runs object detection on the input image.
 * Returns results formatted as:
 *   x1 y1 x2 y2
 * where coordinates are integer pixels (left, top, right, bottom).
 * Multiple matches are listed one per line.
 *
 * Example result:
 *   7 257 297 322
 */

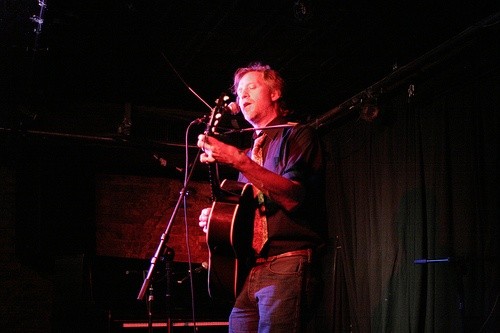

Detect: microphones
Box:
176 261 208 284
211 100 241 134
412 257 450 264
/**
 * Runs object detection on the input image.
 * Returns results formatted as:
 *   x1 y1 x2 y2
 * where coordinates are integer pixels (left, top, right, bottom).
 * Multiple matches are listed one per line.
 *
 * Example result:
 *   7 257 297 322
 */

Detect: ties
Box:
247 134 276 258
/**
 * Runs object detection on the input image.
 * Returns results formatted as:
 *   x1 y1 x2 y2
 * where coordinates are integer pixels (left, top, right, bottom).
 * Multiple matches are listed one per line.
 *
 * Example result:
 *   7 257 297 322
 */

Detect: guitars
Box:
206 93 254 299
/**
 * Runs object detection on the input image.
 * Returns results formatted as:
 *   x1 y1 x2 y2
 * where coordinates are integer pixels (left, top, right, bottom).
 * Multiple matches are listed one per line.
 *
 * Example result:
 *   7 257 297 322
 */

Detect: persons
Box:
197 59 331 333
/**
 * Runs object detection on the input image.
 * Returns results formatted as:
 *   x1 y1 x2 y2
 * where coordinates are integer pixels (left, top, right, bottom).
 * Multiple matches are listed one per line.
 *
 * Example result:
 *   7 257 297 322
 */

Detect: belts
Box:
247 248 315 264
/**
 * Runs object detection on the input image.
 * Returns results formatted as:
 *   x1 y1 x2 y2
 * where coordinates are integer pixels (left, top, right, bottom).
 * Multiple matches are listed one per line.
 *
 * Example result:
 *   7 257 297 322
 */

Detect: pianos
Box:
83 256 232 333
